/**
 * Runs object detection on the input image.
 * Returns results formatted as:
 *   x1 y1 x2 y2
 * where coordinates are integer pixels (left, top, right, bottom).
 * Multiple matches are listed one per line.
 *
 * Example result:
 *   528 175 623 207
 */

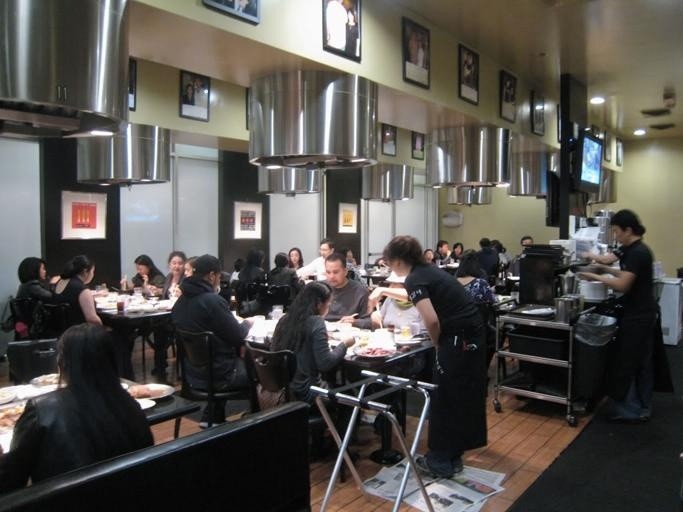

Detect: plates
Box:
579 279 608 300
328 322 421 358
0 373 176 437
93 293 171 313
522 308 553 315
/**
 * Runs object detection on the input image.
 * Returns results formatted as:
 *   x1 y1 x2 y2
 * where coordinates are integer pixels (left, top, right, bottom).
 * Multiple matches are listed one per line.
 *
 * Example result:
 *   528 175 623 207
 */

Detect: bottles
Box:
229 292 238 318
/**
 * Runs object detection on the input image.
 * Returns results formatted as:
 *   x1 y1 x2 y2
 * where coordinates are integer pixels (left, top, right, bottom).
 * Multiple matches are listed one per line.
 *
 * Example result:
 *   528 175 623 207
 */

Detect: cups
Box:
271 304 283 321
511 291 517 304
386 320 420 339
558 274 576 295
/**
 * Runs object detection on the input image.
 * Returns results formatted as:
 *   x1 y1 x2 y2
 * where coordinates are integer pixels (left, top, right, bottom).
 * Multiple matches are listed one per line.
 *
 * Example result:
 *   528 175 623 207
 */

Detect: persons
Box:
463 51 475 87
503 78 513 102
3 323 155 496
424 236 534 311
342 8 361 58
170 253 263 429
577 210 658 424
577 226 626 272
182 83 195 105
14 251 197 385
231 239 428 465
416 38 426 68
407 30 419 66
191 76 208 109
324 1 349 54
382 235 496 479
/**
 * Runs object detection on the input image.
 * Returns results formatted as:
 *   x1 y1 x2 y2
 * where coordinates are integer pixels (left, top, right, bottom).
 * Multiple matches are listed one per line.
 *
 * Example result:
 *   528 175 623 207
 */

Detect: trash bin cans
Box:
573 312 617 390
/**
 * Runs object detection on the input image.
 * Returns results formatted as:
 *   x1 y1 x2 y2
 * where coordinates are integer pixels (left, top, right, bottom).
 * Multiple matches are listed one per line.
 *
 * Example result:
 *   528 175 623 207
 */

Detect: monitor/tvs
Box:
579 131 604 186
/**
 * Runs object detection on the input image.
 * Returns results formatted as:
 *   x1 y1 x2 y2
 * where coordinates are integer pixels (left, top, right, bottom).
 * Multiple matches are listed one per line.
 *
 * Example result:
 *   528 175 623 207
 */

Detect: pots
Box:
554 293 595 323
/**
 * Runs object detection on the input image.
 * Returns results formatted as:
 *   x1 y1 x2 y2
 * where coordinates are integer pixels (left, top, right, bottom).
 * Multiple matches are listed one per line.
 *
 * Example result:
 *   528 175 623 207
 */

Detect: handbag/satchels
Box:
255 381 287 411
0 314 18 334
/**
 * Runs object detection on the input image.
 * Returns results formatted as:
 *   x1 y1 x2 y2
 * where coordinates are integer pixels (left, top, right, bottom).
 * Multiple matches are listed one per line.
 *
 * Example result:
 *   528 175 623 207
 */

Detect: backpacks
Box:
29 300 61 339
238 288 279 319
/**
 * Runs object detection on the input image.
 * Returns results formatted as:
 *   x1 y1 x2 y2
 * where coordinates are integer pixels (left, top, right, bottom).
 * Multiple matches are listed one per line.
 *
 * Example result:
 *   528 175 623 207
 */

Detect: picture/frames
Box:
319 0 363 63
200 0 263 26
400 15 430 91
58 187 107 242
335 201 360 235
176 65 212 122
409 130 425 161
456 40 545 137
128 58 137 113
381 121 398 156
554 104 624 167
231 197 266 245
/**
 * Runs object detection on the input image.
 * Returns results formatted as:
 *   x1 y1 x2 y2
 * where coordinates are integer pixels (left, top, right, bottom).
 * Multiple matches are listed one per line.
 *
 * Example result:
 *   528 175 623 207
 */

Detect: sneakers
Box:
415 455 455 480
198 419 229 429
423 452 464 473
151 361 169 376
606 406 652 425
